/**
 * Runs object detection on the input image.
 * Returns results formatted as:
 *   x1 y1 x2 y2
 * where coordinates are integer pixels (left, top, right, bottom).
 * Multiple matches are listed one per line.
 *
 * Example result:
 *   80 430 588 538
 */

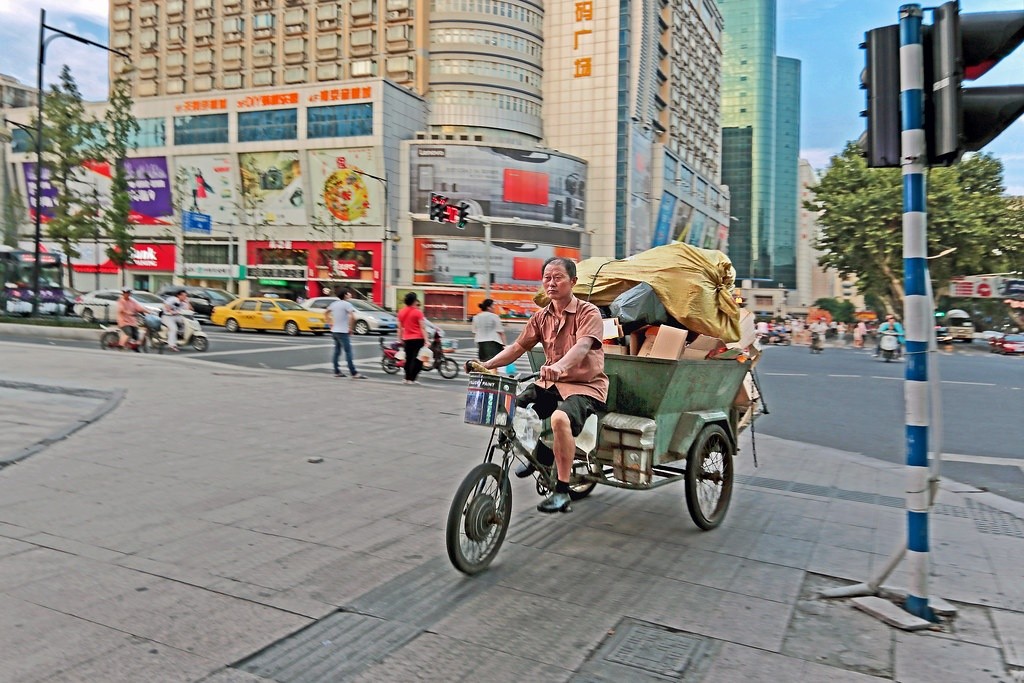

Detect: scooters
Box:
99 311 167 355
150 308 210 352
758 327 904 363
378 331 460 379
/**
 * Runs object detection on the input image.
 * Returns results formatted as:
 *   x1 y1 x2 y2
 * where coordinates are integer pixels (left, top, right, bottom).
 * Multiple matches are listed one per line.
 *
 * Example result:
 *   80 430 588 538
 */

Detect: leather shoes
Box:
537 492 571 513
515 450 553 478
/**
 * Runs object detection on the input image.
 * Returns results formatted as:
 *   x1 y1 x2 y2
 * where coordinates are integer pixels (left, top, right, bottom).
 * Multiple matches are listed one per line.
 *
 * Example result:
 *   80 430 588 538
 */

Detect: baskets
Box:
462 371 518 428
441 338 460 353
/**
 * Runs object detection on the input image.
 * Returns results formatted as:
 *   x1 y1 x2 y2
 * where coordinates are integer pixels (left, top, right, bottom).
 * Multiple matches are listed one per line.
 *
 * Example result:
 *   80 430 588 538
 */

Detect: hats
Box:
122 288 133 293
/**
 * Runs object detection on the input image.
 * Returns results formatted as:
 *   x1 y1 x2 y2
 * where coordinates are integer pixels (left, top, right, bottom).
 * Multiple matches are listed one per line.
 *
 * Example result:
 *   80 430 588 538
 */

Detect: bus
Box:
934 313 945 331
0 244 64 317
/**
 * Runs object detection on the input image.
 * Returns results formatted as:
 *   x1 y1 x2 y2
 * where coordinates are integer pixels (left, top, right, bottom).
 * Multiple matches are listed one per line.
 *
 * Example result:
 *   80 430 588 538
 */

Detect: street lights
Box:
31 7 133 318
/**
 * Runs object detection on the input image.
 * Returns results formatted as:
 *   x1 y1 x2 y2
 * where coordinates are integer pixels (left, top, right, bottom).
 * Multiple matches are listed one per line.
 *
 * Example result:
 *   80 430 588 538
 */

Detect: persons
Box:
872 313 906 361
853 319 867 347
115 287 153 353
395 292 443 385
161 289 195 353
471 299 507 362
757 313 838 351
322 288 368 379
463 257 610 513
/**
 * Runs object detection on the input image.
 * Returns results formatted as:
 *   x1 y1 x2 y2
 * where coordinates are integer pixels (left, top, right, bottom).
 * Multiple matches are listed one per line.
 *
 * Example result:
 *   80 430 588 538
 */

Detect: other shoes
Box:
402 378 420 384
169 345 179 352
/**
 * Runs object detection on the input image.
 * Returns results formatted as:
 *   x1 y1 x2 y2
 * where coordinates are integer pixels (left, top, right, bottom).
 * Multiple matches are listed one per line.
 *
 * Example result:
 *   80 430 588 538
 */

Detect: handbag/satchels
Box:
416 345 435 370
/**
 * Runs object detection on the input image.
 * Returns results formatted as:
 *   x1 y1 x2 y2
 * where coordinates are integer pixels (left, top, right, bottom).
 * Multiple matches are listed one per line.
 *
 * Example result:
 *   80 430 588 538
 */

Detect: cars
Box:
154 285 230 316
936 330 953 345
73 288 169 324
211 294 333 336
996 334 1024 355
62 287 83 317
299 297 342 313
345 298 399 335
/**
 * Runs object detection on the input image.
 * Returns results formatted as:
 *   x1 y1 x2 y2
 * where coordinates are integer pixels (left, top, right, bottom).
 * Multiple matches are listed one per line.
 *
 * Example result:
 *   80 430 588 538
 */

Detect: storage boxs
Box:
603 280 761 404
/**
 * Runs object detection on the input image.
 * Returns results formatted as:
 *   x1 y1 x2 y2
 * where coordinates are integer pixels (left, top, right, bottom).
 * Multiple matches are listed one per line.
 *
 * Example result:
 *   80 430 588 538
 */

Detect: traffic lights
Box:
930 0 1024 164
458 202 470 228
438 196 449 225
429 192 441 221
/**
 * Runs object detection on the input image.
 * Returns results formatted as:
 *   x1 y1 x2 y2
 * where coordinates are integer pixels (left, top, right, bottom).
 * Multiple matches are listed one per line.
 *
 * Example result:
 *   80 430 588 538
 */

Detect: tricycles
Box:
445 345 754 578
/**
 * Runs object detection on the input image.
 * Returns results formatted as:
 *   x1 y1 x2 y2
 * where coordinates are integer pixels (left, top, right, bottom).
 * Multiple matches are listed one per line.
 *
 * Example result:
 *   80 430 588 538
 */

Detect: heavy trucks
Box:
940 309 975 344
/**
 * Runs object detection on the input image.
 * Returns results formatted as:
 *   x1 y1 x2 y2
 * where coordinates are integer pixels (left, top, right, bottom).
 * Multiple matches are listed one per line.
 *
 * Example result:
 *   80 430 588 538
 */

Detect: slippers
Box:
352 374 368 379
334 373 347 377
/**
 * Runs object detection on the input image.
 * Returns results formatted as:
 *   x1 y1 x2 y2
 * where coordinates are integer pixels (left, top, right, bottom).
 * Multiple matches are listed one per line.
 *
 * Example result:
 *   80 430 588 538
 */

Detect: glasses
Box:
889 316 896 319
416 303 421 307
